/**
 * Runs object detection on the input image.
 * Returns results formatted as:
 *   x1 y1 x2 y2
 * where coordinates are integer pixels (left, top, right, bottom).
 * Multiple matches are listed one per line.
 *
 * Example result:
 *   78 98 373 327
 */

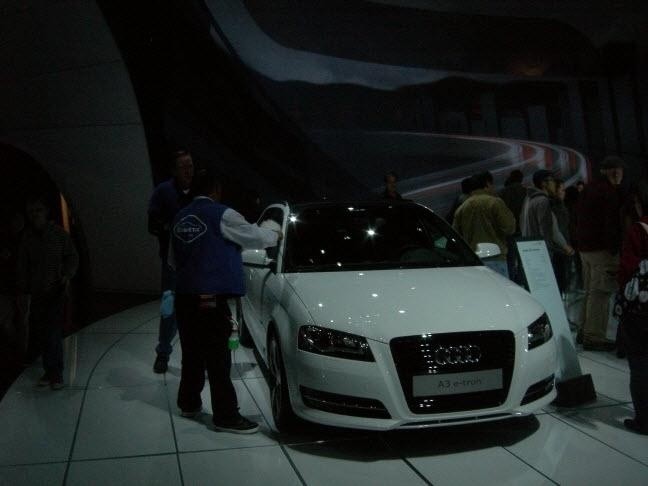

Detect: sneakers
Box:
179 405 204 418
575 333 617 353
152 350 171 375
37 369 66 391
622 416 647 435
213 412 262 435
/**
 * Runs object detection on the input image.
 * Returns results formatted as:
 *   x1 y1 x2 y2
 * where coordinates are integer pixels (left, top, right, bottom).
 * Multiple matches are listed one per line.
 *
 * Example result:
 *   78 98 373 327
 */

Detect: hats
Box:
599 154 632 171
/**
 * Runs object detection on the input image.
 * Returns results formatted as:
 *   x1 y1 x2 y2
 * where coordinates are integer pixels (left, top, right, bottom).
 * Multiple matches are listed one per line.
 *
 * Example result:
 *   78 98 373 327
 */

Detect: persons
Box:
378 171 402 199
166 169 283 434
146 148 202 374
14 195 80 389
614 180 647 435
576 154 627 351
446 168 647 349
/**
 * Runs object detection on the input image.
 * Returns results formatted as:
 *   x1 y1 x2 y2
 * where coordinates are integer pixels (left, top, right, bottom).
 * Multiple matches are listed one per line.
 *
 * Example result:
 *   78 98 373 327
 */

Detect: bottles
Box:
160 290 174 318
228 326 240 351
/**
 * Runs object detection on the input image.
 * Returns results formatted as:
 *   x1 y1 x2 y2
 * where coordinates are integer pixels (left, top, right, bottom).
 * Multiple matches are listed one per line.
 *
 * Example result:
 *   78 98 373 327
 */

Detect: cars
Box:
233 193 566 441
